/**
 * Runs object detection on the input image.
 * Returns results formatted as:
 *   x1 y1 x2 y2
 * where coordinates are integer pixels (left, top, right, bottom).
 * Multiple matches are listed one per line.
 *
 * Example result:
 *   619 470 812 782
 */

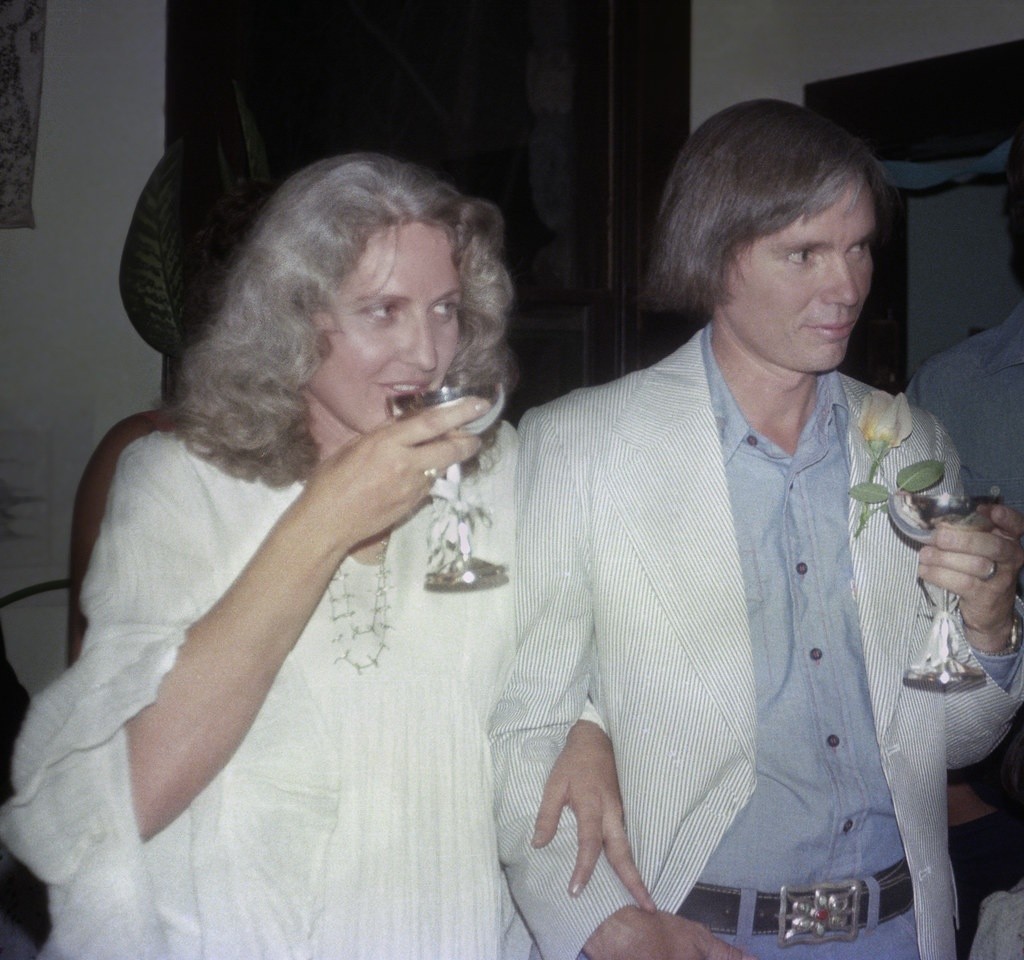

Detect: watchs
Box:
973 610 1024 657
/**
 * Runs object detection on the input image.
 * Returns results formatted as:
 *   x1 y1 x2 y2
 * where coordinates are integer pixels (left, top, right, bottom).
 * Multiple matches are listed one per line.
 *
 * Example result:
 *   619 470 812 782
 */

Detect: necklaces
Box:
327 536 396 679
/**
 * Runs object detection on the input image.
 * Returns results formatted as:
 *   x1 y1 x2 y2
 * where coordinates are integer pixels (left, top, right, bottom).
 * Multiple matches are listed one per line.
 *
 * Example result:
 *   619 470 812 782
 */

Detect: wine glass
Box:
887 486 1006 686
388 379 507 588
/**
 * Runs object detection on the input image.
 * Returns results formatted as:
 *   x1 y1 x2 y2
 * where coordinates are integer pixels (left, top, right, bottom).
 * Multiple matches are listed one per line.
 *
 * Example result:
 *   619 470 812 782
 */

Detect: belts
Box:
677 859 914 946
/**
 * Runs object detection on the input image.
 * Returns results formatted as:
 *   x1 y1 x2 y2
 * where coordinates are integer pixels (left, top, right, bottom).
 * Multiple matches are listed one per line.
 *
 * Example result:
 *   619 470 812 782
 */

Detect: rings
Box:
424 469 438 483
980 561 997 582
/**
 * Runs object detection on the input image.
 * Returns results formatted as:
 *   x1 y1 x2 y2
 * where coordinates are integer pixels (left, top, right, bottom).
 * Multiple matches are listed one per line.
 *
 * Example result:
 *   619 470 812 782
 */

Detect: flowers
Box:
849 389 943 538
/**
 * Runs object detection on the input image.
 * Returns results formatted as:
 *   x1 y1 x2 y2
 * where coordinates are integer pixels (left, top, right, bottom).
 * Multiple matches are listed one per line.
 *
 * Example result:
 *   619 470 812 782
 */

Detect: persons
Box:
0 153 656 959
487 97 1023 959
902 135 1023 959
